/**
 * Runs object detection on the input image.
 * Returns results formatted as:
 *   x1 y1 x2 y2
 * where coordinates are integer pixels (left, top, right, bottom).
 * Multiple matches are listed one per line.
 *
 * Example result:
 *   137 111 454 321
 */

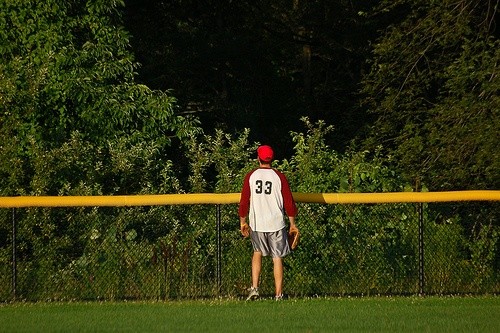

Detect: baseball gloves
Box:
288 230 300 252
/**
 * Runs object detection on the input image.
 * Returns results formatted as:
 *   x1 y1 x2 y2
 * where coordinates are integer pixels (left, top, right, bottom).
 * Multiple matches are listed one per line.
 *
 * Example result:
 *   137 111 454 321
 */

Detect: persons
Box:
238 145 298 302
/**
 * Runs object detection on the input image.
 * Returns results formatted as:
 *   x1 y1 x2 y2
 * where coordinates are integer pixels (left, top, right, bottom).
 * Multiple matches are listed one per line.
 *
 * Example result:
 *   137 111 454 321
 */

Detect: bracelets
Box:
241 222 247 226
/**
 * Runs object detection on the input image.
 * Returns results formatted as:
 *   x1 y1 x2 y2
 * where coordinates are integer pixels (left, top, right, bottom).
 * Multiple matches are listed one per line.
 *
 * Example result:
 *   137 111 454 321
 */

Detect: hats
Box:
257 145 274 161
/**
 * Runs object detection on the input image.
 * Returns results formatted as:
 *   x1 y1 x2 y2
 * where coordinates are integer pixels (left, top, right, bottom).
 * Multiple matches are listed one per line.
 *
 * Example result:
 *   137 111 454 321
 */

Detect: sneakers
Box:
275 293 283 301
245 288 260 303
